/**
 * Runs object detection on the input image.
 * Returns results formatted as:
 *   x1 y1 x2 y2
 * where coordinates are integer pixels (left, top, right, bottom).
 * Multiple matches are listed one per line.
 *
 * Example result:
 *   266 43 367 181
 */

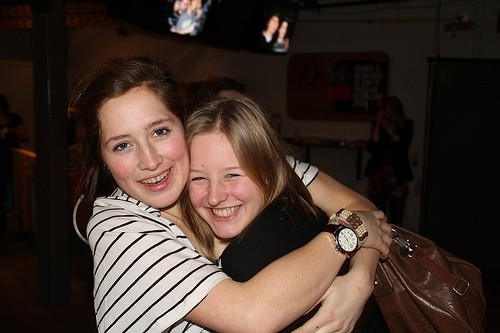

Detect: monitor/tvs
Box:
164 0 299 54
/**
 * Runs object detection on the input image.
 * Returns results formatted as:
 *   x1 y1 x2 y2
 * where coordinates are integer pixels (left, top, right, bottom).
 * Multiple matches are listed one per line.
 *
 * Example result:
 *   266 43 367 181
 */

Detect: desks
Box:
281 135 364 182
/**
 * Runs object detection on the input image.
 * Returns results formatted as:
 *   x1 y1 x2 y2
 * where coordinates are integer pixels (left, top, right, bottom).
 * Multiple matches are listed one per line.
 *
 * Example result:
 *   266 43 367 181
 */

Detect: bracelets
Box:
328 208 368 246
9 127 12 132
387 128 395 134
374 126 380 128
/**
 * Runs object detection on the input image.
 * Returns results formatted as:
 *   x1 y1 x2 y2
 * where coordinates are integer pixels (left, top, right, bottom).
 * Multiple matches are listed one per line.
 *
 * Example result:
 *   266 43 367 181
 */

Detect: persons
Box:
77 56 392 333
368 96 412 228
186 95 391 333
0 94 29 218
261 15 289 53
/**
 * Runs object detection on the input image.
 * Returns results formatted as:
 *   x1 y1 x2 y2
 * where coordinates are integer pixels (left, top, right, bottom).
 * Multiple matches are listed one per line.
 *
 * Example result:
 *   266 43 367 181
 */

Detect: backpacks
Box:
368 220 486 333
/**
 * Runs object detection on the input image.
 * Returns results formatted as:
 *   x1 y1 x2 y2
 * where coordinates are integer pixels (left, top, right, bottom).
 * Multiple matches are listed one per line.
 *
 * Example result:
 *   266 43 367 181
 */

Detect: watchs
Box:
323 224 358 258
